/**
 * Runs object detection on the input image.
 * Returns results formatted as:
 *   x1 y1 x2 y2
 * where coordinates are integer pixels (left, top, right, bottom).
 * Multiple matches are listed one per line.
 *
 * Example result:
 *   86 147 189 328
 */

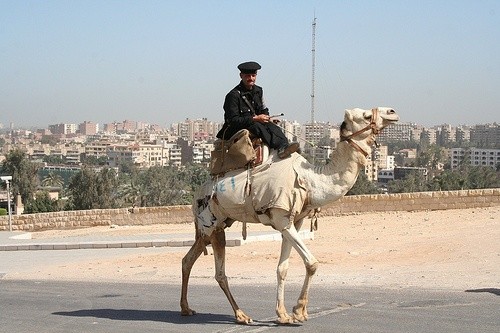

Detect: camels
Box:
179 107 400 325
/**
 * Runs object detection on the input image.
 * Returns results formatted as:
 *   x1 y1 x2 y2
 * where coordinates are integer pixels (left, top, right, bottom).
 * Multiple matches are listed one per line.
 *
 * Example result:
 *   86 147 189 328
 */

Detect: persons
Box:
216 62 304 160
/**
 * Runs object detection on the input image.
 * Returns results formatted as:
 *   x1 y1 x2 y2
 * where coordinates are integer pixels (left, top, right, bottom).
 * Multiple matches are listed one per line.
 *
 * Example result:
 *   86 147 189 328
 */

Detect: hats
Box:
238 62 261 74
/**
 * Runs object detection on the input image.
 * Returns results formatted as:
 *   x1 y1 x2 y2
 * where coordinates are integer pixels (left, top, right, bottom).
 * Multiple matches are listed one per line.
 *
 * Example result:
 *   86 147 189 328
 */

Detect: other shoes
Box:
277 143 299 160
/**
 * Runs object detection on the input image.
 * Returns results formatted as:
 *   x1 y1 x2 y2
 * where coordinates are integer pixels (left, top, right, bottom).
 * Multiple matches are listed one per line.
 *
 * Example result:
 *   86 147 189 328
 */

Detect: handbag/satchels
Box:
210 128 256 175
